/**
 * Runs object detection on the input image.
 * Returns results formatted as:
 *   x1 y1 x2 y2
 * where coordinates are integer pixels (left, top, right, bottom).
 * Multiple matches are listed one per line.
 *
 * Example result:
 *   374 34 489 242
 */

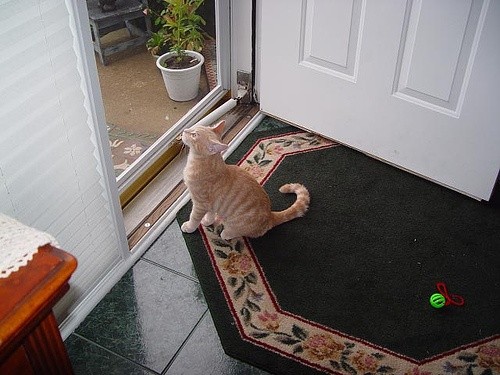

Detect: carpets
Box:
176 125 500 375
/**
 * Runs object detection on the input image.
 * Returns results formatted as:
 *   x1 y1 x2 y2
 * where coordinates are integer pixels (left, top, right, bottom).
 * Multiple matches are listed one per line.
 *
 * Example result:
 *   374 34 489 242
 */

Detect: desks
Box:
0 212 77 375
86 0 155 68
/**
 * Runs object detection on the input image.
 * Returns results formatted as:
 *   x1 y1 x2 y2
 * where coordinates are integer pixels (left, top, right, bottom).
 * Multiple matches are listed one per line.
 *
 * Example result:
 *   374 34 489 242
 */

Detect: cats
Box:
180 120 311 240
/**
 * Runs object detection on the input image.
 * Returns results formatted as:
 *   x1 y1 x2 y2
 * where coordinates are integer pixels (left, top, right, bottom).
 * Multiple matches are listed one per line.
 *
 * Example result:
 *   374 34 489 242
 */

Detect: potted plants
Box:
142 0 208 101
